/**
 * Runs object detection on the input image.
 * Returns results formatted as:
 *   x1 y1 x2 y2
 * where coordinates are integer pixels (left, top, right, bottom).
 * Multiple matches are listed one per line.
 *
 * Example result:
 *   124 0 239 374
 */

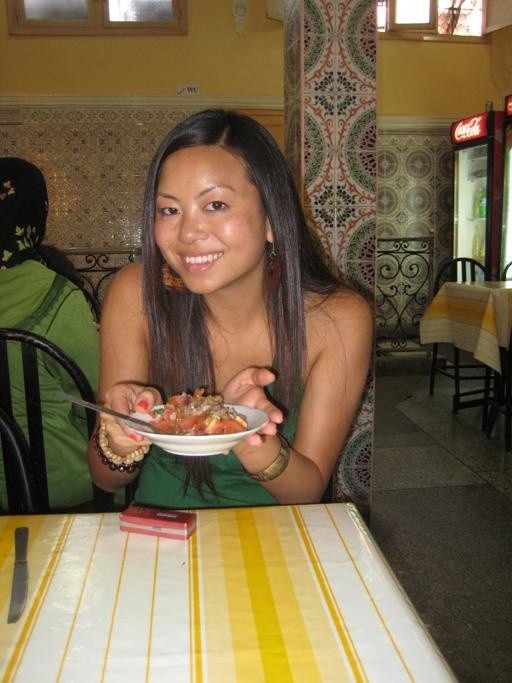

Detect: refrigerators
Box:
450 110 505 282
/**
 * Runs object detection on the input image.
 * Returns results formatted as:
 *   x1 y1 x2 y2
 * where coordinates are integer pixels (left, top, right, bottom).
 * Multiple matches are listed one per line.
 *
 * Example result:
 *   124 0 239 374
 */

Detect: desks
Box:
2 502 464 683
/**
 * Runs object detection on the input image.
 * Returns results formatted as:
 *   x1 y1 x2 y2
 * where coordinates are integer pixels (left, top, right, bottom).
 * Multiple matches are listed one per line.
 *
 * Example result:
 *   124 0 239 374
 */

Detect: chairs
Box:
0 326 134 516
420 256 512 457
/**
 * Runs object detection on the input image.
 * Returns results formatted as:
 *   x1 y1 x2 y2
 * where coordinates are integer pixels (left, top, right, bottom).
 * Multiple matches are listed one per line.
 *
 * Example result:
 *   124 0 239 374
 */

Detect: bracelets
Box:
92 417 152 473
240 432 293 482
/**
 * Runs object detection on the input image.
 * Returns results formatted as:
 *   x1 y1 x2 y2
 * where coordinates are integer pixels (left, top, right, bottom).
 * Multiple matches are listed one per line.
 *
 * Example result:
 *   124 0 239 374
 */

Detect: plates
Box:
124 404 268 456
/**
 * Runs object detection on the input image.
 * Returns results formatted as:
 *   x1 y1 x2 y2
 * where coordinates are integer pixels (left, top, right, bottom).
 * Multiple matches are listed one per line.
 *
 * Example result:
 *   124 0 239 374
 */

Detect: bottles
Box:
473 184 487 258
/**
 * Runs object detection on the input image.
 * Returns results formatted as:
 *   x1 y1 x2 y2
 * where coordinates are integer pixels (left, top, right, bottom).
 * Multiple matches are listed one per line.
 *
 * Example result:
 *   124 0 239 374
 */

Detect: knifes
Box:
8 524 29 623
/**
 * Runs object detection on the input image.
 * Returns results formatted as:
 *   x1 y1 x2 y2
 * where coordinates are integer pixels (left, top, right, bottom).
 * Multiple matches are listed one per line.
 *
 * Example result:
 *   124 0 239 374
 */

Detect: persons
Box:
89 108 377 512
2 153 101 512
